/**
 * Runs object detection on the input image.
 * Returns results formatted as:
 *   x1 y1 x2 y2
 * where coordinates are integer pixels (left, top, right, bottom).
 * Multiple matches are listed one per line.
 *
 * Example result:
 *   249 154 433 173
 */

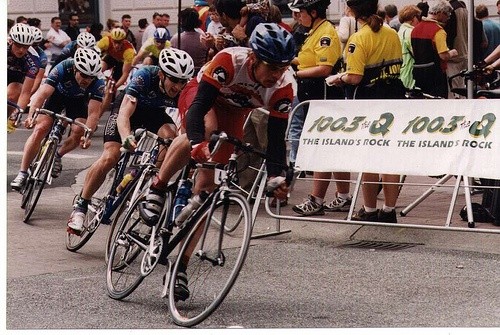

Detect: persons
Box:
170 8 209 77
7 23 40 120
200 0 282 58
378 0 500 99
132 28 172 65
16 13 170 61
10 48 105 192
29 26 48 96
286 0 353 216
98 27 135 118
138 23 298 298
67 48 194 233
328 0 403 223
50 32 100 72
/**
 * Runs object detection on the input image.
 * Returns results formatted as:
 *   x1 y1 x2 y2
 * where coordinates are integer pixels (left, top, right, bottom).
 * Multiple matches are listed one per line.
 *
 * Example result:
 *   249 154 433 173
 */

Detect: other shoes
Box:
272 196 288 207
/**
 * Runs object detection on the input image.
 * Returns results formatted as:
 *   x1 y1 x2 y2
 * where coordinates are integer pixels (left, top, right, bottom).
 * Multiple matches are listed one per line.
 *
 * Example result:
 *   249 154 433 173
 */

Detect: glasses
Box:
255 55 290 73
415 14 421 22
164 73 188 85
155 39 166 44
78 70 96 80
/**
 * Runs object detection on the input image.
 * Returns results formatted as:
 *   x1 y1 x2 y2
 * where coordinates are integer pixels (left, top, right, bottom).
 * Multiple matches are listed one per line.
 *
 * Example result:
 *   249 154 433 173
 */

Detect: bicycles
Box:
105 118 294 328
102 65 119 97
449 63 500 190
7 98 92 223
66 124 191 271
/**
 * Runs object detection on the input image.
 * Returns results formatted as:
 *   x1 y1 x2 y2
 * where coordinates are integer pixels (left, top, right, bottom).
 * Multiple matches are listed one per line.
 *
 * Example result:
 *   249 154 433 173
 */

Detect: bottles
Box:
173 177 193 226
175 190 208 227
115 169 138 193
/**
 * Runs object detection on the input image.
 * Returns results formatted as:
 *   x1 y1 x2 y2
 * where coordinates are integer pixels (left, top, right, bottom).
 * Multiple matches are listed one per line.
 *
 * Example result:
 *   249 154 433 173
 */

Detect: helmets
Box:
110 28 126 40
154 28 170 41
249 22 296 64
76 32 96 49
30 26 43 42
159 48 195 79
74 47 103 76
9 23 35 45
291 0 319 8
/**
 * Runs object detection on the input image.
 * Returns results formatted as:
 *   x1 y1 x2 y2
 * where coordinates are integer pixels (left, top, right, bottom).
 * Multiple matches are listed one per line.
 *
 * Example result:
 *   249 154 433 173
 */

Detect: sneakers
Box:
323 191 356 211
67 209 86 232
7 115 17 134
50 156 62 178
292 193 326 216
343 205 379 223
10 171 27 190
377 208 397 223
50 123 66 144
140 175 164 224
162 271 189 299
